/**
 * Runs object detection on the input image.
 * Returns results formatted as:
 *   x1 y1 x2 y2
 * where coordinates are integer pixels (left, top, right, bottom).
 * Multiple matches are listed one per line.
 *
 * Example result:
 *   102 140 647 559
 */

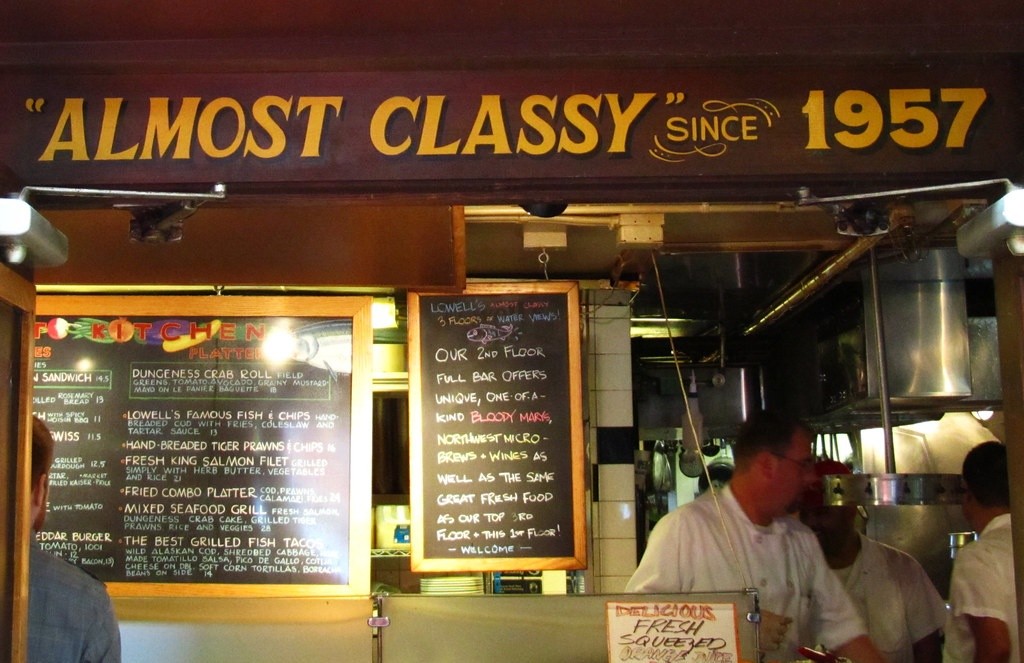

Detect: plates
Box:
631 315 711 338
418 576 486 595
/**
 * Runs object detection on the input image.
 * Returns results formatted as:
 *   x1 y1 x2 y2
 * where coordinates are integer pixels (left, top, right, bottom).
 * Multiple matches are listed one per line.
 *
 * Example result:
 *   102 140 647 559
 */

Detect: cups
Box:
375 504 409 548
948 530 976 559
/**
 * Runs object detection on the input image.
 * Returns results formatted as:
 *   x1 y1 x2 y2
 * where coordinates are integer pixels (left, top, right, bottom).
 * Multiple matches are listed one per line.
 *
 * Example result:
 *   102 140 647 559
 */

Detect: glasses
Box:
770 451 815 473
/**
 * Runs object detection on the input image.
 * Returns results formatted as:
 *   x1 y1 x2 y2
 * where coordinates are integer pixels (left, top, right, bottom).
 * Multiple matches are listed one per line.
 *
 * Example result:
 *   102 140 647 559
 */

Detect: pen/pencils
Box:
807 590 811 606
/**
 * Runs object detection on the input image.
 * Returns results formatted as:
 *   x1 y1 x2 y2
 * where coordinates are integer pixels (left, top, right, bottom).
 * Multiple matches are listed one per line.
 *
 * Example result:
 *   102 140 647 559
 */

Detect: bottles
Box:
682 368 705 450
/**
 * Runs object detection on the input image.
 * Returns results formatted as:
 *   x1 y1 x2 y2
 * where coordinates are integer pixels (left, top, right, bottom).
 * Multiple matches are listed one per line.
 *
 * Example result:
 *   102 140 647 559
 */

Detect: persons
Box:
26 415 121 662
802 459 950 663
626 408 880 662
942 441 1020 663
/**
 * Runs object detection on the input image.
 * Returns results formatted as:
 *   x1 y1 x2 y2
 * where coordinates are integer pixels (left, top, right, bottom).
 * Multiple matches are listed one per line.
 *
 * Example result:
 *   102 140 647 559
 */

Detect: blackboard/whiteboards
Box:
30 295 372 597
407 281 585 573
